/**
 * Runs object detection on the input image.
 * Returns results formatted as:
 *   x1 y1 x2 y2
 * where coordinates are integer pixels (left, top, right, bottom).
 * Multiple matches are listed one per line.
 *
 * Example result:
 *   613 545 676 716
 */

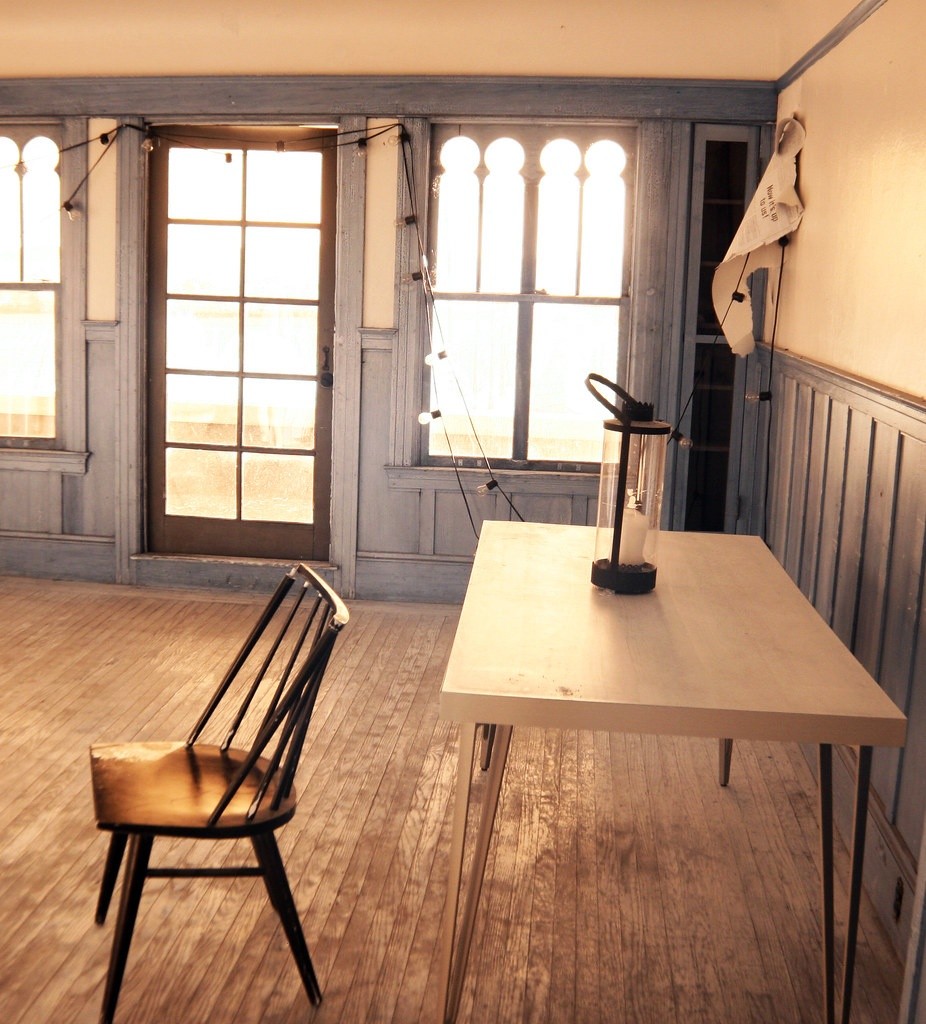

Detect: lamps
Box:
585 373 670 592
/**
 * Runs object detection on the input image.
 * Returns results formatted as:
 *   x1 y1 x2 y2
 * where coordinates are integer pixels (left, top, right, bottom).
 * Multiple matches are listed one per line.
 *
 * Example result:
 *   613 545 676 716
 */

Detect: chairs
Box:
90 562 350 1024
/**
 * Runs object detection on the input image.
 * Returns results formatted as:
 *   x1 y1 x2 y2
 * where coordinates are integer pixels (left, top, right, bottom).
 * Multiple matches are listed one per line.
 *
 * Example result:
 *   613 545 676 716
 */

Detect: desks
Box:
440 521 908 1024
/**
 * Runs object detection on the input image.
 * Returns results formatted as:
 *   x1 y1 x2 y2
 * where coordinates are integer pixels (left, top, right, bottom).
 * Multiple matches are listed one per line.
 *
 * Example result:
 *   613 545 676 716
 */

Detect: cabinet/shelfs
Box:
673 124 760 532
